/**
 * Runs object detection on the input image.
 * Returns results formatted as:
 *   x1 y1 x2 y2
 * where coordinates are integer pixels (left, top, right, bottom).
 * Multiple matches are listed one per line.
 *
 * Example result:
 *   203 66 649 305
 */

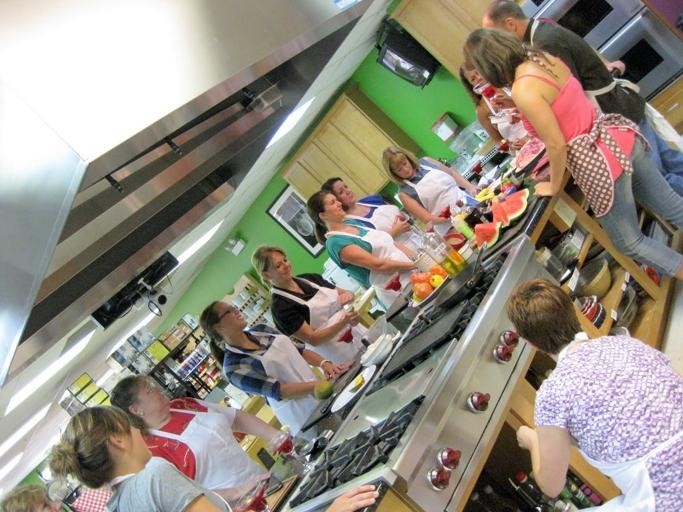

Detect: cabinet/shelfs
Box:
463 143 501 178
236 366 325 472
276 83 423 203
193 272 273 363
644 73 682 135
528 165 682 353
464 322 623 510
146 325 225 400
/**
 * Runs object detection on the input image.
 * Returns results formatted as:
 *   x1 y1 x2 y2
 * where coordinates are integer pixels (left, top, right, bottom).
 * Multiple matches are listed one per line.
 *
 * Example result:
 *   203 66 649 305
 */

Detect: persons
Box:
0 1 682 512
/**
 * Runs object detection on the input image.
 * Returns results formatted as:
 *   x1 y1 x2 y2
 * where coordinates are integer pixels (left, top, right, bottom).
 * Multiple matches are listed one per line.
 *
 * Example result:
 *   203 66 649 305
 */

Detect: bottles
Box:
469 466 603 511
400 213 468 282
451 201 490 239
341 303 359 328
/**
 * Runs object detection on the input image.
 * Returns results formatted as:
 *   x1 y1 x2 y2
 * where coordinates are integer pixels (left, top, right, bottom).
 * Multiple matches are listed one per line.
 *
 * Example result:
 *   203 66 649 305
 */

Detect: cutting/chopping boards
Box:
253 474 298 512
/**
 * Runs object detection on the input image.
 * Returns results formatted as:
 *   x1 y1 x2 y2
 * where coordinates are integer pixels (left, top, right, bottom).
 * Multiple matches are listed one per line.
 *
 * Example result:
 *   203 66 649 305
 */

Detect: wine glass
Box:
470 159 493 183
265 431 317 474
496 138 521 162
336 327 368 354
382 272 418 310
473 79 509 116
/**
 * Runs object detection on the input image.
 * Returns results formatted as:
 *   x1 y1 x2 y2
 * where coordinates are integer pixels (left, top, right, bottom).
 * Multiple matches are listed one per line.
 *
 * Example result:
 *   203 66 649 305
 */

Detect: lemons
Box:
314 380 333 400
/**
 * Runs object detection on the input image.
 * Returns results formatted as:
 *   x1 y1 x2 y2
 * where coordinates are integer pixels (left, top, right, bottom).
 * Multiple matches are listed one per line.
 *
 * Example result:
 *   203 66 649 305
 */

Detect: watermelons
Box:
473 187 531 252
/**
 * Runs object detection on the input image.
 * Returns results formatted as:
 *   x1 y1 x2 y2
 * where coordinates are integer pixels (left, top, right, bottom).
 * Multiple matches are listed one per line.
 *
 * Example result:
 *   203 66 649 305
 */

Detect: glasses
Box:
216 305 238 323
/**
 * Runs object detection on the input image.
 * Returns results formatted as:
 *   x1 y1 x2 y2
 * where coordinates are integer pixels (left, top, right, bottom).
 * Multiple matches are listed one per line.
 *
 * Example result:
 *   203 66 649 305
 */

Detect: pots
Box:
432 242 491 310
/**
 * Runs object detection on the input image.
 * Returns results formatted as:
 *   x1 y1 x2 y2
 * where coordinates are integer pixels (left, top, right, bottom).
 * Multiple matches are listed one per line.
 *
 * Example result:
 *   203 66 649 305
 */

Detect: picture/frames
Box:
265 183 325 258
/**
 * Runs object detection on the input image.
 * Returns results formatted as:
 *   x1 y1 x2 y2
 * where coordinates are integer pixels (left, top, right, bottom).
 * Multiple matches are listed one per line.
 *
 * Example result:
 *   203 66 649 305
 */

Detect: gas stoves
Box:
285 394 508 511
374 252 547 392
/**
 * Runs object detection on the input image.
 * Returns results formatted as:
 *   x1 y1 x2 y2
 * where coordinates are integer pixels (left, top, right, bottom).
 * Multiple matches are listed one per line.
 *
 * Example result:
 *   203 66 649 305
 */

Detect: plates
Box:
513 145 545 174
574 293 606 329
359 331 395 366
483 187 517 214
328 366 379 411
410 273 448 309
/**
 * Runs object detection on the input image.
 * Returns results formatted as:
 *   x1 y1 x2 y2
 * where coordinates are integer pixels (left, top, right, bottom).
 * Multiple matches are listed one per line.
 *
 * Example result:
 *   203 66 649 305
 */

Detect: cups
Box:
534 247 564 277
234 484 270 512
445 232 467 247
437 205 458 223
410 247 442 273
459 245 475 259
397 212 412 230
421 220 443 241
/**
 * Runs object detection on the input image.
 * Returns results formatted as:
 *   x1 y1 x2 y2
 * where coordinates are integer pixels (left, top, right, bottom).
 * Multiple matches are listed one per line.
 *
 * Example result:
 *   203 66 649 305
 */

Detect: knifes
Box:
319 365 363 413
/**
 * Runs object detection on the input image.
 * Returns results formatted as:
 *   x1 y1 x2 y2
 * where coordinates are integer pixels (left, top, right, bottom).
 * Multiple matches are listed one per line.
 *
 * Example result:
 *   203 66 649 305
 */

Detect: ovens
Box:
521 1 681 109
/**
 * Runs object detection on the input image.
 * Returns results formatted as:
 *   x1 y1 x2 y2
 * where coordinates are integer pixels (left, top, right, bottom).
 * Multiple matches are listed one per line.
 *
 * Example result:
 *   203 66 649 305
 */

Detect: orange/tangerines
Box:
410 265 447 303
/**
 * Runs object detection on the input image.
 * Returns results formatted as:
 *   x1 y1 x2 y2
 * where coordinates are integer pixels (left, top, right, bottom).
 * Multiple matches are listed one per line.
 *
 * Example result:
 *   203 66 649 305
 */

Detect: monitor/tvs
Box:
376 29 436 88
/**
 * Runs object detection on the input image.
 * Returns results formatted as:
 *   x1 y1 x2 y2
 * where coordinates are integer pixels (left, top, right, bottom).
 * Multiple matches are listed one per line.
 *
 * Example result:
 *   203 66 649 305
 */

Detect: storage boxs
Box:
428 112 460 142
58 372 112 417
106 314 200 377
465 119 490 142
448 126 484 161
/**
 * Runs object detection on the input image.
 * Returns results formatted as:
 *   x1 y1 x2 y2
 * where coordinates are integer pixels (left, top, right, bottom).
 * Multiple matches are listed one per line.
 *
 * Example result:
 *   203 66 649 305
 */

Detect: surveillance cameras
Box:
149 287 166 305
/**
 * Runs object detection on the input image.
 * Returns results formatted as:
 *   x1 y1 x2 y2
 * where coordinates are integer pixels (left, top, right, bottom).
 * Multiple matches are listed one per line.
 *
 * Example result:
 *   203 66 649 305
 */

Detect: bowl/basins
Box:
606 285 644 346
576 257 614 299
